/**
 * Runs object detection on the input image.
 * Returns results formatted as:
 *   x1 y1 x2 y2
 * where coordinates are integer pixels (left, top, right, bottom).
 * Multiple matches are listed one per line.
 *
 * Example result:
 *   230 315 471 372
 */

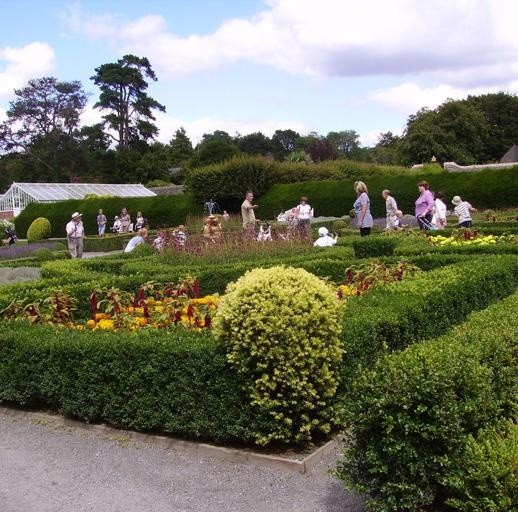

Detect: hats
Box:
114 216 119 220
452 196 462 205
318 227 329 237
71 212 83 220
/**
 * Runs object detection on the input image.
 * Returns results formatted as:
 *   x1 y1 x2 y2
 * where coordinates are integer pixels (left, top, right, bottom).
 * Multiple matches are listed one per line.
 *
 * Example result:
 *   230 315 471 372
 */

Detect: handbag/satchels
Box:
257 225 272 241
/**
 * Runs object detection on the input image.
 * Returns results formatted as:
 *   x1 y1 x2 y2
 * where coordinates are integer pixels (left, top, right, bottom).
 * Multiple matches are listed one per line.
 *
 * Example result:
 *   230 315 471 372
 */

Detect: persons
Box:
451 196 478 228
97 207 186 253
66 212 85 258
353 181 373 237
1 230 15 247
241 190 338 246
223 211 230 222
382 180 448 230
